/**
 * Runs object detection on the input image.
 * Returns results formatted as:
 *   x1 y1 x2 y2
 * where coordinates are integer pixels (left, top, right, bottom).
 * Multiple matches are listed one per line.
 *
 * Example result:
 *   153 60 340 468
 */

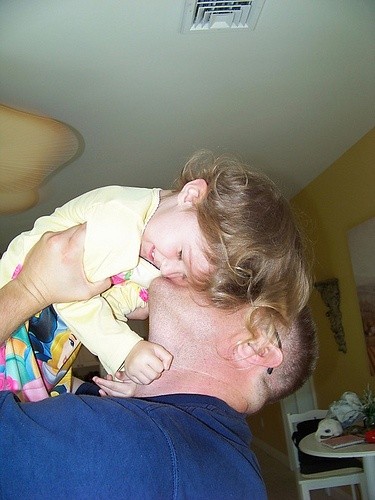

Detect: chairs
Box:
287 409 367 500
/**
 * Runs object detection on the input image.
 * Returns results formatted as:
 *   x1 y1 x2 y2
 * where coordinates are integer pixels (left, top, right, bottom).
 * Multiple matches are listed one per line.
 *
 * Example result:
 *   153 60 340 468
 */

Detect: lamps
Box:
1 104 80 214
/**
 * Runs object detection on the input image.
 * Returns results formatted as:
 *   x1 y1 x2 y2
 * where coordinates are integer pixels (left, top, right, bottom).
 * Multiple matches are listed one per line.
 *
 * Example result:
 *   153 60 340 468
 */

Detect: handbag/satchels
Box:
292 419 362 474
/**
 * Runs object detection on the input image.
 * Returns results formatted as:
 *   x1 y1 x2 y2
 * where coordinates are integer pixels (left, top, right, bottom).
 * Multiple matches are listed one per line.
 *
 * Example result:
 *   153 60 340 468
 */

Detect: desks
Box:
298 423 375 500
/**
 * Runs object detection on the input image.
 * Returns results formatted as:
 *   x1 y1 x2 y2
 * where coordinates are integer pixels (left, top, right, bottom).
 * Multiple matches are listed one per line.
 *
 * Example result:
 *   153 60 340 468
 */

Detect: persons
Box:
0 273 318 500
0 156 315 404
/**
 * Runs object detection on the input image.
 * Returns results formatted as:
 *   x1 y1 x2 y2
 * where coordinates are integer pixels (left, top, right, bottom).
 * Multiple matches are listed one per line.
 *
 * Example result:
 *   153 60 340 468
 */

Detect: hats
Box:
315 420 344 442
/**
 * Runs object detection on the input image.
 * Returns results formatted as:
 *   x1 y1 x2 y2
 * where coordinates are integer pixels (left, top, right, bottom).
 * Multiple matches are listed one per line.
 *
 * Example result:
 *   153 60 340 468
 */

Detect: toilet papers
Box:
316 417 343 441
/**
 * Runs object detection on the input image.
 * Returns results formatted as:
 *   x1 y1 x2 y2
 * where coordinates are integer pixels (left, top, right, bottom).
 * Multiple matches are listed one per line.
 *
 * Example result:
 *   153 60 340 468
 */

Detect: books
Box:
323 433 362 449
